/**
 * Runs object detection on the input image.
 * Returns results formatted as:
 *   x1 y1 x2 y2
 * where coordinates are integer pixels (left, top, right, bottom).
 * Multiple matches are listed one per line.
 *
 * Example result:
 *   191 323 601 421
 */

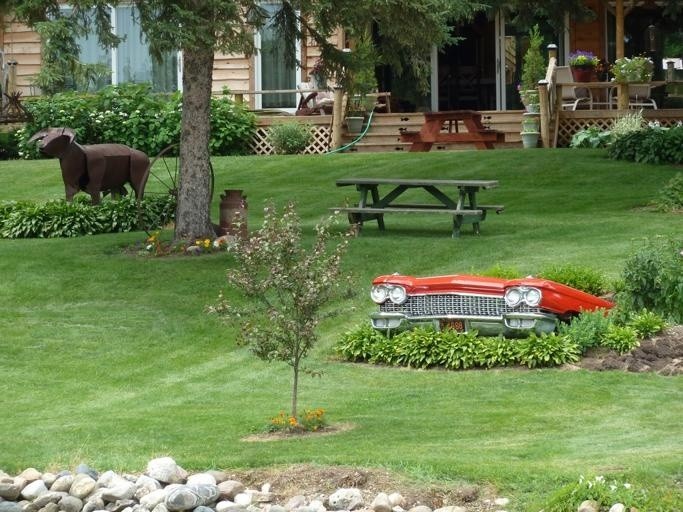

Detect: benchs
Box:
328 202 506 238
400 132 505 154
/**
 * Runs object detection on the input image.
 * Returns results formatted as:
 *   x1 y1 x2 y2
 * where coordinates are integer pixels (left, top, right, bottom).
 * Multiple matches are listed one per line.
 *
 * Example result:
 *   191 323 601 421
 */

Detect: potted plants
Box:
518 23 547 148
569 55 597 79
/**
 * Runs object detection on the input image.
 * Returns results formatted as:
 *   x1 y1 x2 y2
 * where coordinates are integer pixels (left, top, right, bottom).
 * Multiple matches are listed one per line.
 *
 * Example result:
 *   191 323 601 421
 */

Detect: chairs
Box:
293 82 337 117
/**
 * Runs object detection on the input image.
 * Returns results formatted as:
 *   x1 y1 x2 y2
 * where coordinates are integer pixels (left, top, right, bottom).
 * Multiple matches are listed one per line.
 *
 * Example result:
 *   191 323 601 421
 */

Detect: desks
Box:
410 110 487 152
337 177 498 237
